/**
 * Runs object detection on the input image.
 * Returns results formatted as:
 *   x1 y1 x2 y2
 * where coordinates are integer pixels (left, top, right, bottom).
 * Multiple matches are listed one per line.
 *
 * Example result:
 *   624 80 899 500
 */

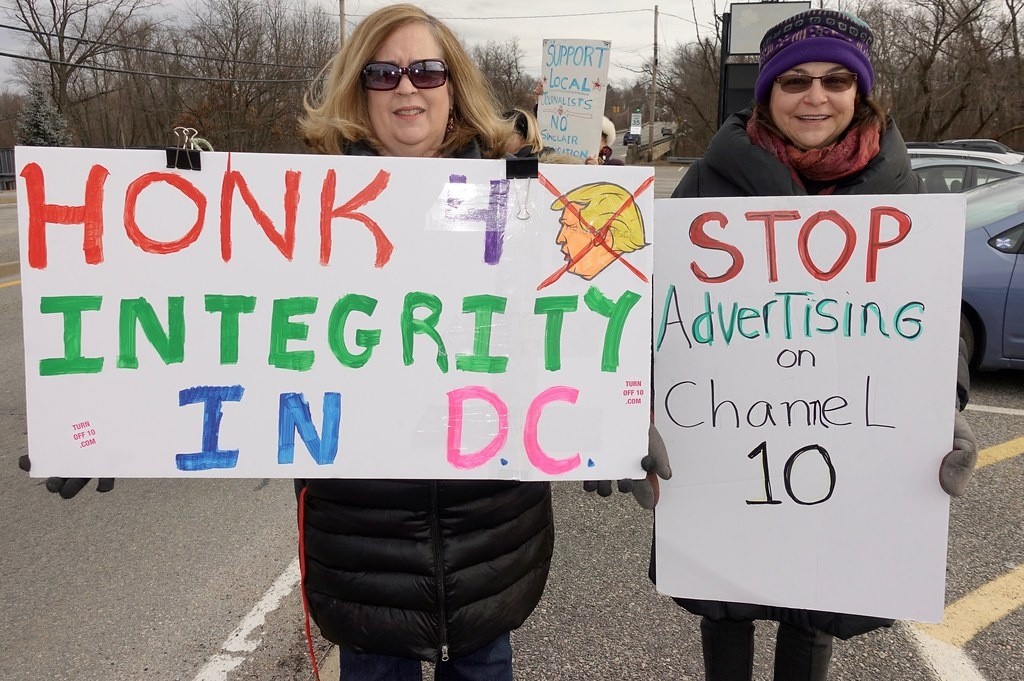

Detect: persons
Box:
500 108 555 158
19 4 657 681
533 83 624 166
629 11 977 681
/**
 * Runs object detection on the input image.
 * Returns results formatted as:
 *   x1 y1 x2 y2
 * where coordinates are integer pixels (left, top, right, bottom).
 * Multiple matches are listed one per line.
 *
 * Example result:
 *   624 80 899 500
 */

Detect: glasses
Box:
360 58 450 91
773 72 857 93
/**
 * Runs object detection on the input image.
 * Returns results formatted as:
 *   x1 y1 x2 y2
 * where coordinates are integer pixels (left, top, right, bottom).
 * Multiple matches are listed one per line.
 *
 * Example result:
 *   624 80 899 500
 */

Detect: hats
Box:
500 111 528 138
600 115 616 147
754 8 875 103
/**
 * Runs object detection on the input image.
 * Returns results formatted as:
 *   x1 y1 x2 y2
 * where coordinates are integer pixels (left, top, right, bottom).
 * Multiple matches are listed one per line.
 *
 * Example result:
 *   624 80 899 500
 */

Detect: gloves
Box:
18 455 115 500
583 422 673 510
939 390 978 498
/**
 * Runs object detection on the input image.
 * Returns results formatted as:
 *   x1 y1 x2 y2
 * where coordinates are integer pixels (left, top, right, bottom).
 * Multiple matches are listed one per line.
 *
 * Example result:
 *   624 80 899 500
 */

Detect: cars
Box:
661 127 676 136
623 131 641 145
896 137 1024 382
